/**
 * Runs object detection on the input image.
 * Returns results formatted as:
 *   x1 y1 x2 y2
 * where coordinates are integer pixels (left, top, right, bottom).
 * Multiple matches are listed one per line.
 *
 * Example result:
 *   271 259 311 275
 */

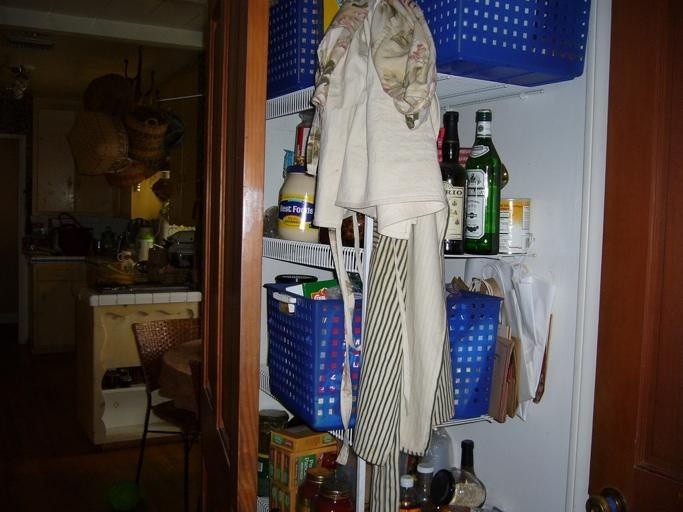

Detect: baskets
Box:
405 0 592 88
261 280 503 432
266 0 326 104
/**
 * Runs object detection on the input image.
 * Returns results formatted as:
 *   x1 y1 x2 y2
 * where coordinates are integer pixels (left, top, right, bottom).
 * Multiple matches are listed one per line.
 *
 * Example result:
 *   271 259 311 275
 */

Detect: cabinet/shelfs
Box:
29 261 116 355
259 73 537 512
26 96 202 223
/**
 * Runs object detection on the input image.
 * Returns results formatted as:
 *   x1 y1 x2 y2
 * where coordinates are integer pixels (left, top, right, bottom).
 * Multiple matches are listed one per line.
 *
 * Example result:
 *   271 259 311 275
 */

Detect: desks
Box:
159 338 202 420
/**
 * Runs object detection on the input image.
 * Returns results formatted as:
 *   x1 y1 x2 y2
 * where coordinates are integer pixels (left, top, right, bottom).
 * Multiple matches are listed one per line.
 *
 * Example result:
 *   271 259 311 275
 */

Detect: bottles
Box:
459 440 476 482
439 112 465 254
431 468 485 506
465 108 499 252
399 476 420 512
417 465 434 511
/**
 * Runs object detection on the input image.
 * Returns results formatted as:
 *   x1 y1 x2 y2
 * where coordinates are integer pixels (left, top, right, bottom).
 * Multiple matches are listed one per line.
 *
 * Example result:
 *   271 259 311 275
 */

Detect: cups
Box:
117 251 131 262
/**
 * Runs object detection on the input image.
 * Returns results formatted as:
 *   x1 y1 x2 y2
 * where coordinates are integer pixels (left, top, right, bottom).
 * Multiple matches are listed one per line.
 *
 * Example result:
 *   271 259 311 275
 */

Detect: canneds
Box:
256 409 352 512
498 198 531 255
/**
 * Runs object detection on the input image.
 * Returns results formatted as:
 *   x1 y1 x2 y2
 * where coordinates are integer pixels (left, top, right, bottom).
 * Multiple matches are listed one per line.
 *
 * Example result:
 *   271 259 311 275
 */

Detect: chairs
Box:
131 318 203 512
188 359 203 419
